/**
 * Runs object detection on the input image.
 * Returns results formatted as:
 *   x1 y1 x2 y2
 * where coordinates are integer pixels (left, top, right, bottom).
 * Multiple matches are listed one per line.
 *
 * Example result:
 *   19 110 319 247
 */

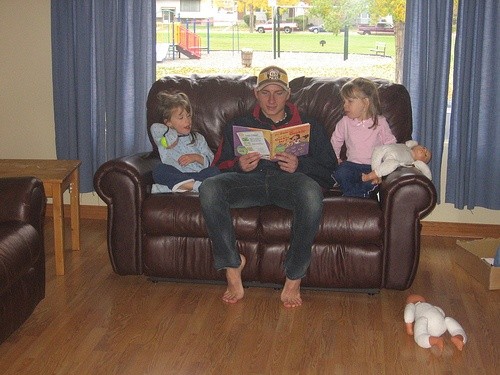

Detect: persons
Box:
404 294 467 352
199 65 338 308
329 76 396 197
361 139 432 183
149 92 220 193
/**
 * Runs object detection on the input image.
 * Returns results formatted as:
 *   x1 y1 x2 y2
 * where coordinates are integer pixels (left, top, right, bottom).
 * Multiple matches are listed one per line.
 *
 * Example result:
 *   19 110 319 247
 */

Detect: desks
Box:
0 158 82 276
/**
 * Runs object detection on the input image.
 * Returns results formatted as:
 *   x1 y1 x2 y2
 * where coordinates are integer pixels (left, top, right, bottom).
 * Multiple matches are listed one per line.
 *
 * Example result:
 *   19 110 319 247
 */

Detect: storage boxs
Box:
455 237 500 291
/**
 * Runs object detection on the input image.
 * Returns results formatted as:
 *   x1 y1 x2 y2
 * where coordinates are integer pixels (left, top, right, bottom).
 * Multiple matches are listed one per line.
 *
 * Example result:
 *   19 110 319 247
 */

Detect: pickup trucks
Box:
255 19 298 34
357 22 395 36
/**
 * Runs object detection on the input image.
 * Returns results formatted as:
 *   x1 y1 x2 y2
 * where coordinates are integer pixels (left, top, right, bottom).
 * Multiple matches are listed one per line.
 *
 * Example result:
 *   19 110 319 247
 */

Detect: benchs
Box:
369 42 386 56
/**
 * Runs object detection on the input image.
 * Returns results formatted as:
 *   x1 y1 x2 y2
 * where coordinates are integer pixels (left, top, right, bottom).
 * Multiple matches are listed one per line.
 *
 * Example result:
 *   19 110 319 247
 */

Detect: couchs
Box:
0 177 47 345
92 73 437 296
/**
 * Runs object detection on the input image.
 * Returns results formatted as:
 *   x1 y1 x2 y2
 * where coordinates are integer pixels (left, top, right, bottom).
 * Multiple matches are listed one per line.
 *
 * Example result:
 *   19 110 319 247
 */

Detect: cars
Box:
309 24 326 33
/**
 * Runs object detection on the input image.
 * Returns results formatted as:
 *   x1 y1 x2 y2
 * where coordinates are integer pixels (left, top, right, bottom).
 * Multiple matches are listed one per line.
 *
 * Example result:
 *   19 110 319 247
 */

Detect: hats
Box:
257 66 289 92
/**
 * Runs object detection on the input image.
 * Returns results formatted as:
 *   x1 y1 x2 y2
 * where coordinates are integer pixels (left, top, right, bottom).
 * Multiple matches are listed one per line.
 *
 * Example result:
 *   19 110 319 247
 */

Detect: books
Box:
233 124 310 162
